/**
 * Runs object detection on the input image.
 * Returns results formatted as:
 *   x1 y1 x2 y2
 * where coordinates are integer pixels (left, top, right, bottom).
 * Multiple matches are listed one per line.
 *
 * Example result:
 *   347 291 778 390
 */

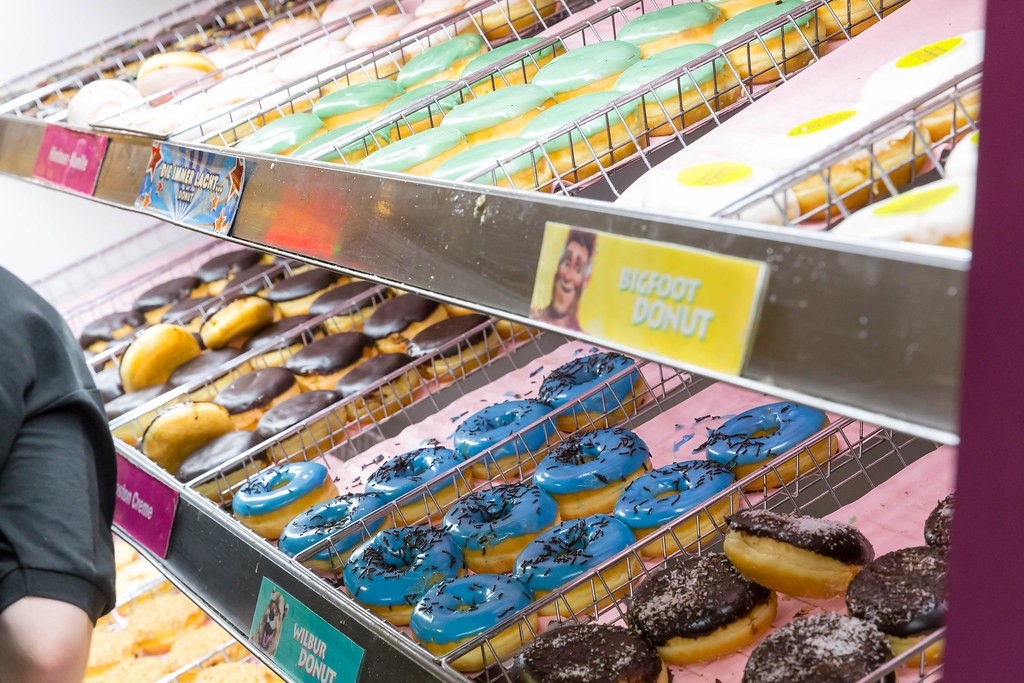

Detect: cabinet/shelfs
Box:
0 0 1024 683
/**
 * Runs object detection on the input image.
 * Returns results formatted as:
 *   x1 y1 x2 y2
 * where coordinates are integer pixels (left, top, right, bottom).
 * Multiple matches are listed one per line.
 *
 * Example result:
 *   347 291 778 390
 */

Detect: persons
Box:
0 264 118 683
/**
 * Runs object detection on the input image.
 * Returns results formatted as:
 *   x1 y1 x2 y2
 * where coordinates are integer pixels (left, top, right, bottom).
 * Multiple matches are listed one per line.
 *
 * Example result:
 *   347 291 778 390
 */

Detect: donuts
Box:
79 249 538 503
1 0 983 261
230 351 950 683
